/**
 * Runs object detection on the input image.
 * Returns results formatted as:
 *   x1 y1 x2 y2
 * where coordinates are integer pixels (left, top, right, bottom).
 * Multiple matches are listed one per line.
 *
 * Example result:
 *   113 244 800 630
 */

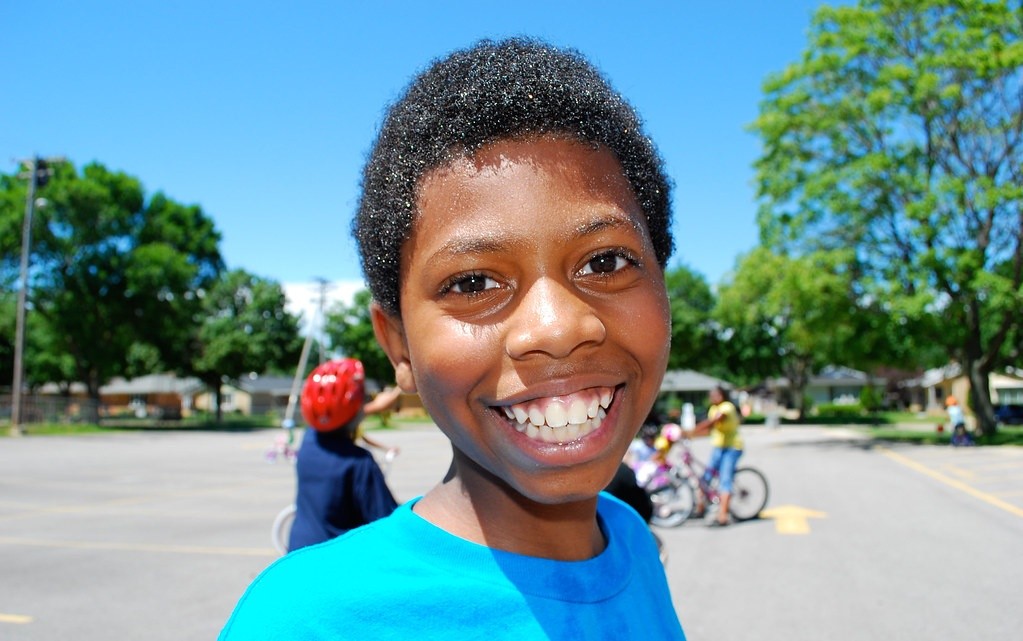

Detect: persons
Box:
285 355 403 555
213 38 687 641
946 395 970 446
603 383 744 569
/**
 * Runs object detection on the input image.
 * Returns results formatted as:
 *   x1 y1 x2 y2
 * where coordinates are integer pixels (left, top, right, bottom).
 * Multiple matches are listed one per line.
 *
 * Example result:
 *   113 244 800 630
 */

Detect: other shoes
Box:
710 519 726 527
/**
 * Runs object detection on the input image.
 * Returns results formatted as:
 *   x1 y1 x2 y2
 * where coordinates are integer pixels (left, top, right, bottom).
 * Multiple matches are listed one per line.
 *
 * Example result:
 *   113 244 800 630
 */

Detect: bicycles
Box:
641 424 770 530
276 445 398 556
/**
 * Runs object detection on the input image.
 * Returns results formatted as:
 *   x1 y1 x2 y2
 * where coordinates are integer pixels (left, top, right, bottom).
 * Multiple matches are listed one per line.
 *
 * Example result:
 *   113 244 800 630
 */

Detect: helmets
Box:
300 358 365 430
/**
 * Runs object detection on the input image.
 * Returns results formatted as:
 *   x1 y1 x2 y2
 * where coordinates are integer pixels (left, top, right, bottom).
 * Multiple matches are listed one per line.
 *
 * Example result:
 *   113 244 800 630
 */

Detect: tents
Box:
659 366 733 394
